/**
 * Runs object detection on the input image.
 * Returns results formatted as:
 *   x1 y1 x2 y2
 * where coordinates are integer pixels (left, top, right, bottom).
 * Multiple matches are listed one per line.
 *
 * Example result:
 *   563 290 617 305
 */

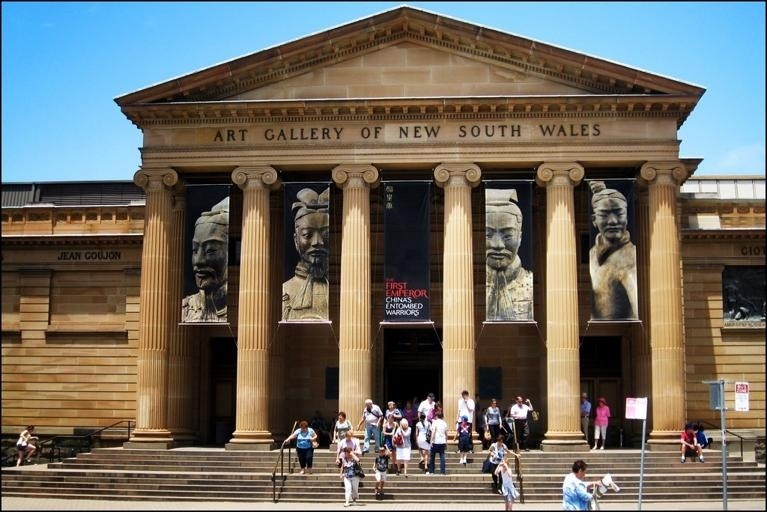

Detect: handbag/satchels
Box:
589 496 600 511
484 429 491 441
426 429 432 443
482 460 493 474
393 435 403 445
311 440 319 448
354 461 365 478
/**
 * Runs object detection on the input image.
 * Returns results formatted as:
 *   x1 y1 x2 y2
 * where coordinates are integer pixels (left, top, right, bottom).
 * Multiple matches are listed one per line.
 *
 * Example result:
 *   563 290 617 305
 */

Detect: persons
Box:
485 188 533 321
182 197 230 322
16 426 40 466
282 188 330 319
560 460 601 510
680 423 710 462
579 394 611 451
589 180 637 320
331 391 534 511
285 421 318 475
